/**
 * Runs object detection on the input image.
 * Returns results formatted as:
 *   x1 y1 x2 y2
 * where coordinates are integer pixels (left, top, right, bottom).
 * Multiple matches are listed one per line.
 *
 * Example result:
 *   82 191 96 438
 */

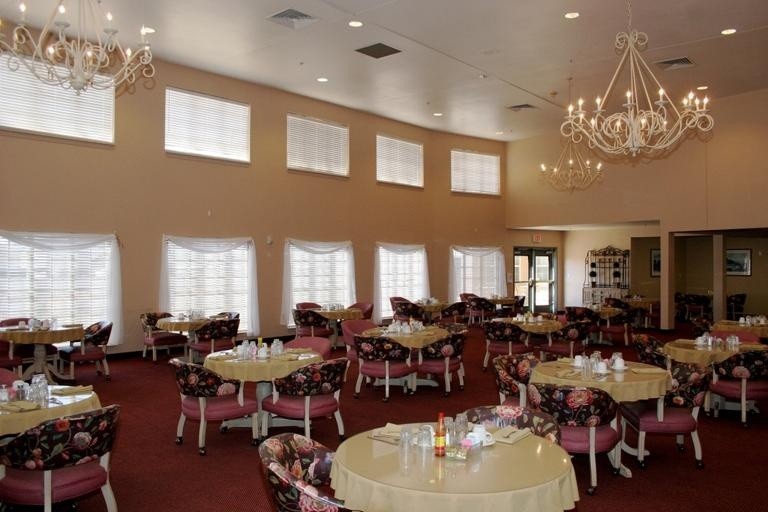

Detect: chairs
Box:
479 305 615 372
280 299 374 356
491 353 542 411
527 379 624 497
339 317 468 401
258 431 359 511
618 361 715 469
137 308 241 364
169 355 259 455
456 404 561 447
0 314 116 391
259 355 352 441
1 403 125 510
387 293 529 330
630 314 768 426
600 296 745 333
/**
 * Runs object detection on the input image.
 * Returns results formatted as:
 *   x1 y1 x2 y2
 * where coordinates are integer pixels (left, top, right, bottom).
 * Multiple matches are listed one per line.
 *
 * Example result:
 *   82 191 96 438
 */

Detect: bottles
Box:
432 455 446 493
435 413 446 456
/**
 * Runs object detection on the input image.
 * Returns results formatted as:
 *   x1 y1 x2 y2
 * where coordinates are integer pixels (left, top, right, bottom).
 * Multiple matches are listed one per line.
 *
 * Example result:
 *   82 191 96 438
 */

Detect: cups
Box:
398 449 413 475
177 311 207 321
570 351 628 377
415 448 432 483
693 333 740 350
380 315 426 337
512 311 547 324
1 373 52 406
399 427 412 448
233 337 285 362
613 371 625 383
739 314 768 326
595 374 607 382
321 303 345 311
447 446 495 482
445 414 496 450
19 318 56 331
417 426 432 449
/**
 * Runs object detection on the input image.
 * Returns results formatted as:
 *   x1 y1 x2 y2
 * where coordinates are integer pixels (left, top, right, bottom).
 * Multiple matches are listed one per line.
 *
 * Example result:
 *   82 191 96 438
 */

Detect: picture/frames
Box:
650 248 661 277
726 249 752 277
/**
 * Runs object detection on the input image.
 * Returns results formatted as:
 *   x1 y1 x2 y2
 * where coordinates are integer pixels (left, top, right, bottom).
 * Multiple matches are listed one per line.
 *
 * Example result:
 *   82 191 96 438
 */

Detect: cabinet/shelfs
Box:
581 245 631 305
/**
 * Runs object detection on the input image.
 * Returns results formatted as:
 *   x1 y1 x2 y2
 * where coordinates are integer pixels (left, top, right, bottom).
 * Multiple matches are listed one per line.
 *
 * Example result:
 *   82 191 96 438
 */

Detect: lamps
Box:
0 0 154 91
560 0 713 158
539 79 603 192
